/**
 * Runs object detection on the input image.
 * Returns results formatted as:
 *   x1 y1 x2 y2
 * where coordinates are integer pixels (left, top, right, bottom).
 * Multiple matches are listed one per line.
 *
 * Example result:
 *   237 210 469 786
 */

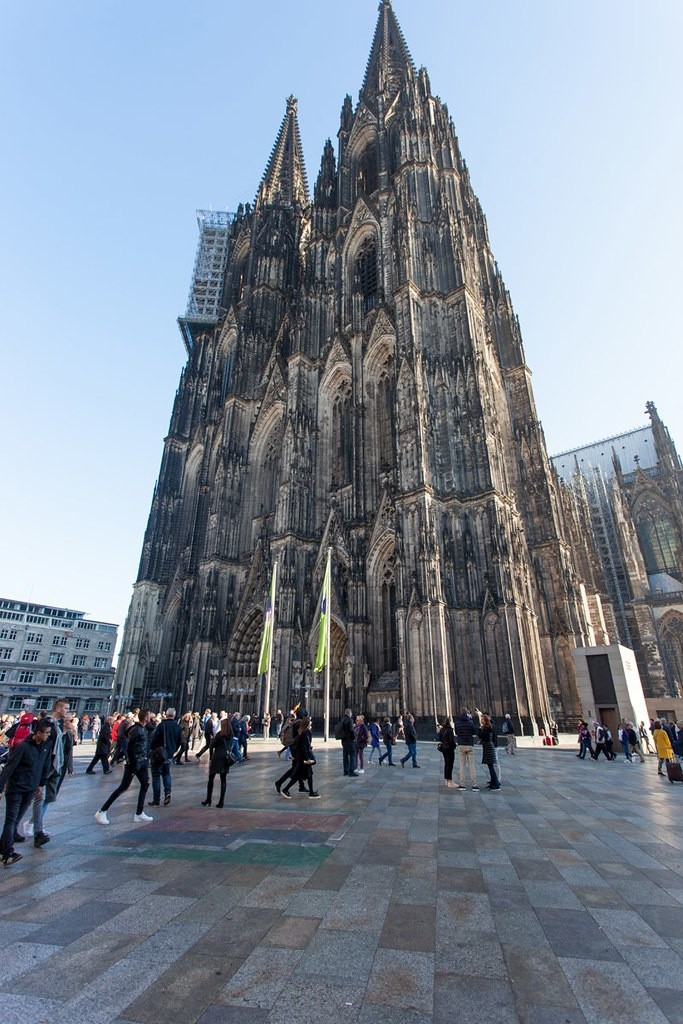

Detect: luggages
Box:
660 749 683 785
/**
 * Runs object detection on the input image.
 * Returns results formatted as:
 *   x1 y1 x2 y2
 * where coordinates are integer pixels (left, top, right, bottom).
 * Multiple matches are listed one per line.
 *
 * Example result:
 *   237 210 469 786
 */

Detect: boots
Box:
201 789 213 808
216 789 228 809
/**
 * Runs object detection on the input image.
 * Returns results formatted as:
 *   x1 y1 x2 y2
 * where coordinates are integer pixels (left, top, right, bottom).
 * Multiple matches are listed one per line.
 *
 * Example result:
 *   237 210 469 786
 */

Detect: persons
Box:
439 718 460 788
353 715 369 774
281 717 322 800
476 715 502 792
94 708 153 824
379 716 397 767
202 718 234 808
454 706 481 792
148 708 182 806
400 715 421 769
1 720 55 867
15 723 51 848
340 708 360 777
22 699 74 837
273 708 312 795
367 718 387 765
0 547 682 778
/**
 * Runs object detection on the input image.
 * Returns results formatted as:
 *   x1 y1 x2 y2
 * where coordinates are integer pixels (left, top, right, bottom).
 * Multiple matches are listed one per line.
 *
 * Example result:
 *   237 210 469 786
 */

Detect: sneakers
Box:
273 780 282 794
165 792 171 806
32 832 51 848
444 779 451 786
24 821 34 837
297 783 310 794
457 786 467 791
3 852 22 867
485 785 501 792
135 812 154 822
147 800 160 806
280 789 292 799
12 829 26 843
471 786 480 792
94 809 107 825
309 790 322 799
447 780 460 788
